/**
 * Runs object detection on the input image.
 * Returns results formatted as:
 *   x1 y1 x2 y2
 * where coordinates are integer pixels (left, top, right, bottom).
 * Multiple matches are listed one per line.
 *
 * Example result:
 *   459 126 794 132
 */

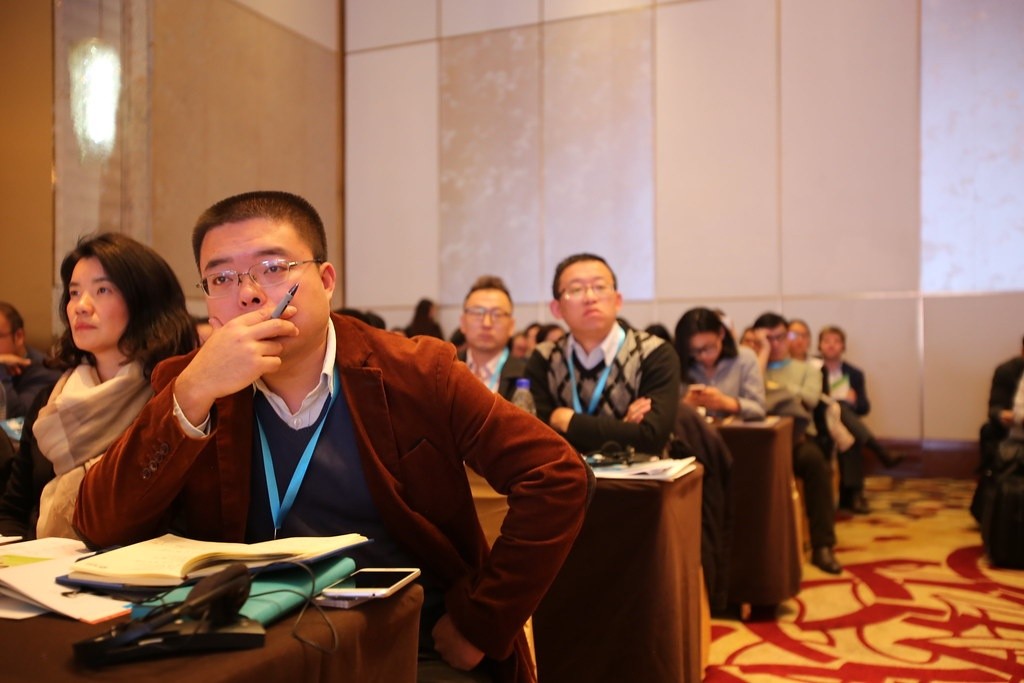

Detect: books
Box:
0 532 373 634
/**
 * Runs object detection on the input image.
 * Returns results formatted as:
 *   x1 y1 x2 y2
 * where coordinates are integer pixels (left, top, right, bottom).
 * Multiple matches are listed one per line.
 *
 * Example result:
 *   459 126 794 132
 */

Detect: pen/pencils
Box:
271 284 302 321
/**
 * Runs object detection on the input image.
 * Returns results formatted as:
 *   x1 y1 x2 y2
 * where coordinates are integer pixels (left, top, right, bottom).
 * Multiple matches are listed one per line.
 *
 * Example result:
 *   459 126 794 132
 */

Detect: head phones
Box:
584 441 633 466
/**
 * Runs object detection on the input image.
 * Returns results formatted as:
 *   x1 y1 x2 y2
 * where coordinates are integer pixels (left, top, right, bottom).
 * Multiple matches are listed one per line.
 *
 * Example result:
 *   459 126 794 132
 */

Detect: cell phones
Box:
321 568 421 598
690 385 707 395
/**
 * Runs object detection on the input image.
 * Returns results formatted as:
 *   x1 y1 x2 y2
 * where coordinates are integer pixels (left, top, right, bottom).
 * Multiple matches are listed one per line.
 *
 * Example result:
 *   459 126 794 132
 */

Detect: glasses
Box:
765 332 789 344
690 335 719 357
195 259 323 299
556 283 616 303
464 308 511 322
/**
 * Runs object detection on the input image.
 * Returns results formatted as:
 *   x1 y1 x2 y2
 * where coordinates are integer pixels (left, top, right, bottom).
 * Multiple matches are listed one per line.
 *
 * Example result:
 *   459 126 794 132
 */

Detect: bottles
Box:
512 379 537 419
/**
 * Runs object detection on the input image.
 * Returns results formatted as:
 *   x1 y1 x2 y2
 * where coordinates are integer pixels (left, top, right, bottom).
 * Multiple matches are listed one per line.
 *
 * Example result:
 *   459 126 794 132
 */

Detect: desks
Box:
703 414 796 626
0 583 423 683
462 455 703 683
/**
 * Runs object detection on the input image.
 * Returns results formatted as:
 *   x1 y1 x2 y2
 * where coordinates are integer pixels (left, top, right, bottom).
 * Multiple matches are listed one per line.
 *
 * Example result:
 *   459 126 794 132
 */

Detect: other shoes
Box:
839 496 869 512
812 546 841 574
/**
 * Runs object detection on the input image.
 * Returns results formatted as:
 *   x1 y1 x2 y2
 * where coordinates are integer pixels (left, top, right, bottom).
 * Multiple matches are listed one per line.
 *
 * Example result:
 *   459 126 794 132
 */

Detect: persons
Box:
70 192 595 683
337 299 465 346
818 326 872 514
641 306 767 420
457 277 531 405
0 301 50 418
523 253 682 455
12 232 197 539
508 324 564 354
989 338 1024 474
750 314 842 573
788 319 831 396
